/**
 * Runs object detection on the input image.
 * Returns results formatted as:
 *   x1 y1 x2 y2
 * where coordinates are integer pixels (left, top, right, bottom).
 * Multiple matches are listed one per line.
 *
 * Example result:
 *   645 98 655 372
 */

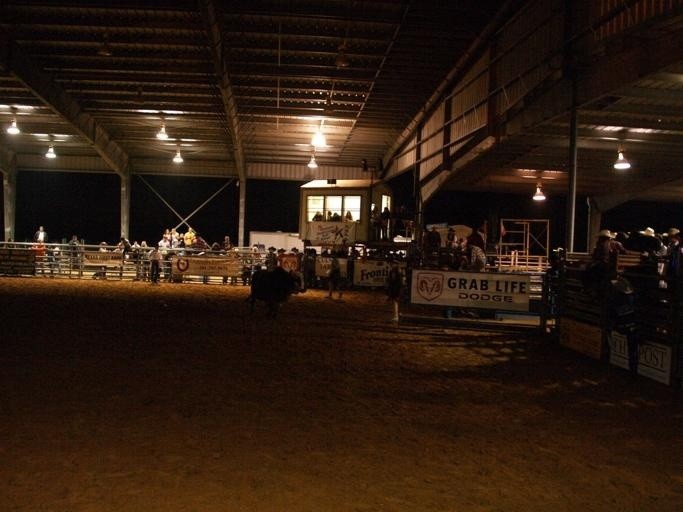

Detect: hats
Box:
268 246 299 253
610 276 635 294
592 229 618 239
637 227 680 237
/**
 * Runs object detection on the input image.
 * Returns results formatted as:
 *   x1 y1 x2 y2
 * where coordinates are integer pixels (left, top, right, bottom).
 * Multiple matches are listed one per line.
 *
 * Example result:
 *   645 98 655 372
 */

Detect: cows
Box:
241 266 306 321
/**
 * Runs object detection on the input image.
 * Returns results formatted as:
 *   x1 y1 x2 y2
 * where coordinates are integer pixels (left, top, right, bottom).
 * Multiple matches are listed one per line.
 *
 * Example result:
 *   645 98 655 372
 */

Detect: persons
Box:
301 247 358 300
98 237 162 282
32 226 81 277
585 227 683 322
212 237 299 286
428 227 487 317
159 228 202 280
371 205 422 300
312 211 353 222
7 238 16 249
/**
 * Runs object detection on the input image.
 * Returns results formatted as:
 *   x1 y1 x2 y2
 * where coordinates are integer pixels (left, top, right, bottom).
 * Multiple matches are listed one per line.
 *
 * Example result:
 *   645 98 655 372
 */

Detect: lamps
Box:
613 149 631 169
172 147 184 164
44 145 56 159
6 114 20 136
308 153 318 169
532 180 546 200
156 125 169 141
